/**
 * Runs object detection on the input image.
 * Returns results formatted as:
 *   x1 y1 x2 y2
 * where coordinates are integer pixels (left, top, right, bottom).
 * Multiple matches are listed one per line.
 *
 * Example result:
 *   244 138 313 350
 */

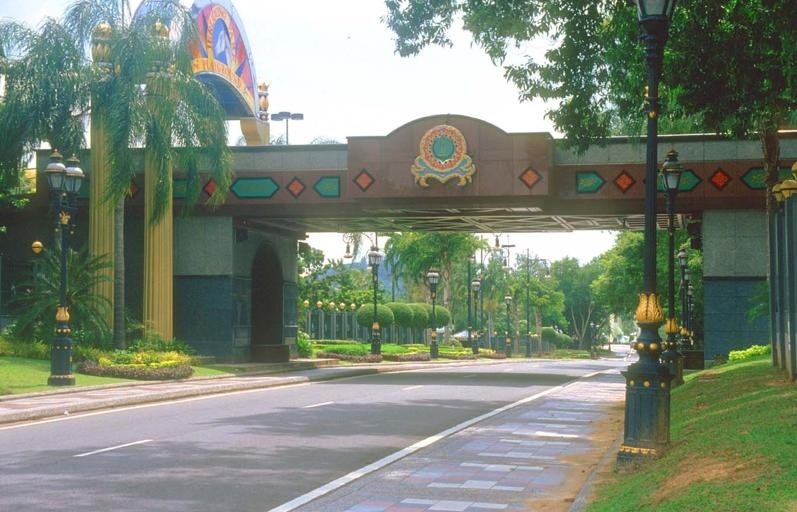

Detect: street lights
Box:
29 149 87 387
342 229 388 358
467 278 483 356
267 109 307 145
420 268 445 358
499 294 516 359
613 0 697 463
522 246 552 359
